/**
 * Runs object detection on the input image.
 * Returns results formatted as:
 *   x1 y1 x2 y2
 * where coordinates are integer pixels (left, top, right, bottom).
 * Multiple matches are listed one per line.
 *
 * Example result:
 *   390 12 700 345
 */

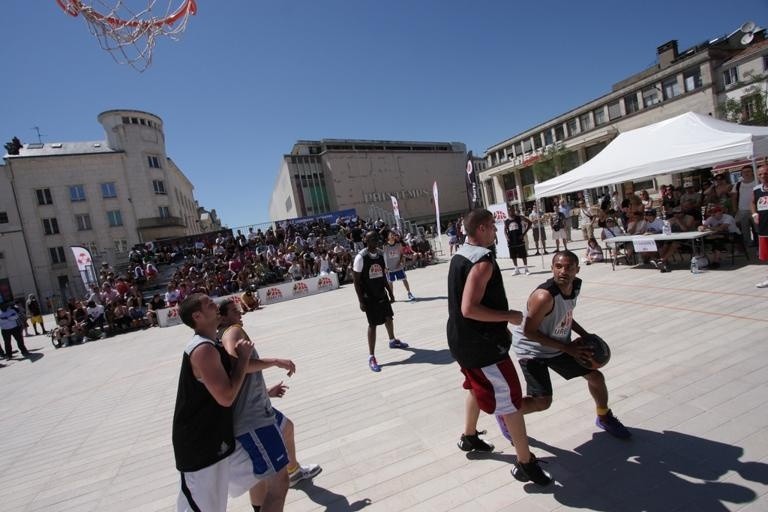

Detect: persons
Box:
445 214 468 256
171 293 296 512
382 231 415 303
446 207 550 487
49 214 439 347
576 164 768 288
503 207 533 275
0 292 49 368
495 250 632 447
211 296 323 512
550 205 567 252
352 230 409 372
528 204 549 255
559 199 574 242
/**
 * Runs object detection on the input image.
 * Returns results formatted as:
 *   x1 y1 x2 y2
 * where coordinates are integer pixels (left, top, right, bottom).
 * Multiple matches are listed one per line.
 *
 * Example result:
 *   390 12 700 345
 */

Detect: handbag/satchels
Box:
582 208 594 220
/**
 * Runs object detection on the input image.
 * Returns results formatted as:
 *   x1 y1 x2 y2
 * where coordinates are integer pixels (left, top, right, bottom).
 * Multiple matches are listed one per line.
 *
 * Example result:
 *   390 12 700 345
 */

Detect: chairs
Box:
600 231 633 272
714 221 750 265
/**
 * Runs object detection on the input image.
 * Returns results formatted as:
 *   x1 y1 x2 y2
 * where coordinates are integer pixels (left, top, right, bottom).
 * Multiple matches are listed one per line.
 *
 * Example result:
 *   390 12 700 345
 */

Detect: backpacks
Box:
552 213 562 232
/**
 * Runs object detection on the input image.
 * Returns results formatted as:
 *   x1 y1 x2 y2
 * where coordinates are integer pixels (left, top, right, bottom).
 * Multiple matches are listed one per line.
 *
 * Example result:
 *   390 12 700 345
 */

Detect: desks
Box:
603 231 713 272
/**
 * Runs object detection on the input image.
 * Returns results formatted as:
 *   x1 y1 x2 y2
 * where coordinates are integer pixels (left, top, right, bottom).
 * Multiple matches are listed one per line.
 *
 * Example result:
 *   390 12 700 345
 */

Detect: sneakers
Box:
390 339 408 348
288 462 322 488
408 294 415 300
512 248 570 277
625 254 721 272
510 452 551 487
369 357 381 372
0 329 49 368
55 323 158 348
756 280 768 288
457 429 495 451
596 409 632 438
496 415 513 442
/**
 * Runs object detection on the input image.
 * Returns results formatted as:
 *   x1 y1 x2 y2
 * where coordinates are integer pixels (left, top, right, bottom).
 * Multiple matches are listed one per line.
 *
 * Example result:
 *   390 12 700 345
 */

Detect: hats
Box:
606 217 614 223
709 206 722 214
683 182 693 188
643 208 656 215
671 206 684 214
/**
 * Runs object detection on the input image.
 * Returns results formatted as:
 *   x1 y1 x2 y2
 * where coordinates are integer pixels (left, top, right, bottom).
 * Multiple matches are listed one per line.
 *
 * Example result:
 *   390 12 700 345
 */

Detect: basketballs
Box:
575 334 611 370
250 284 256 291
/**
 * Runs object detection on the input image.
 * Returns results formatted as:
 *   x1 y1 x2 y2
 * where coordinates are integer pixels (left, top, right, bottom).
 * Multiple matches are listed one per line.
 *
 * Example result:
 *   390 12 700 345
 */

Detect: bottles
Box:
691 257 698 273
662 221 671 236
